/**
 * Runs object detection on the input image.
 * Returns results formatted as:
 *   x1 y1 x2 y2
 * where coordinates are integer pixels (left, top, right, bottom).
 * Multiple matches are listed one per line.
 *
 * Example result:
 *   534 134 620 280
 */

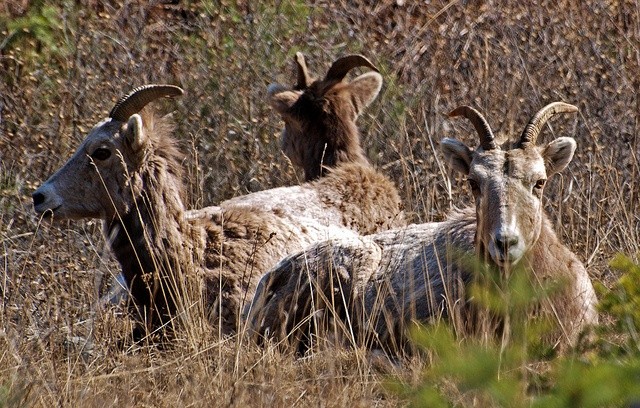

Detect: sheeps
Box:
241 101 600 368
33 84 367 331
176 50 406 236
